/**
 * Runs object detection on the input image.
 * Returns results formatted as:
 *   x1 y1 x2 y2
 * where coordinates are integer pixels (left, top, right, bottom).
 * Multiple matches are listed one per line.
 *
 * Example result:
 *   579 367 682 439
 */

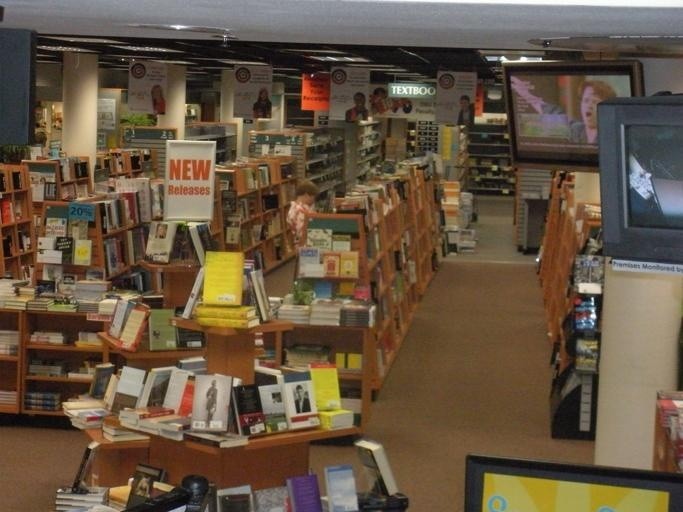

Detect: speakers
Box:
0 29 37 146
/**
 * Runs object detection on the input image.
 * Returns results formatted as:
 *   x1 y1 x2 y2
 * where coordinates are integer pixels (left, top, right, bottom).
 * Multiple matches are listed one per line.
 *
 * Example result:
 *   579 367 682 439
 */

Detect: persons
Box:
149 84 164 115
456 93 475 125
250 88 271 118
510 74 619 142
343 91 368 123
370 87 404 114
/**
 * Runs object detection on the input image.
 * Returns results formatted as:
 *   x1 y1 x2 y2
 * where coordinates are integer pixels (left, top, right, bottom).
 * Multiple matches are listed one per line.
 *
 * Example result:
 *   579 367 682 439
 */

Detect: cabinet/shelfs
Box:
537 173 602 441
54 309 409 512
467 123 509 197
0 119 468 429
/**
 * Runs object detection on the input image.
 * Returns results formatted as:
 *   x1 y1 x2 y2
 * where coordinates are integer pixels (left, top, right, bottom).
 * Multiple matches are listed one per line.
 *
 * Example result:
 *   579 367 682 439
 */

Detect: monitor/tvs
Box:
595 95 683 266
464 452 683 512
501 57 645 172
